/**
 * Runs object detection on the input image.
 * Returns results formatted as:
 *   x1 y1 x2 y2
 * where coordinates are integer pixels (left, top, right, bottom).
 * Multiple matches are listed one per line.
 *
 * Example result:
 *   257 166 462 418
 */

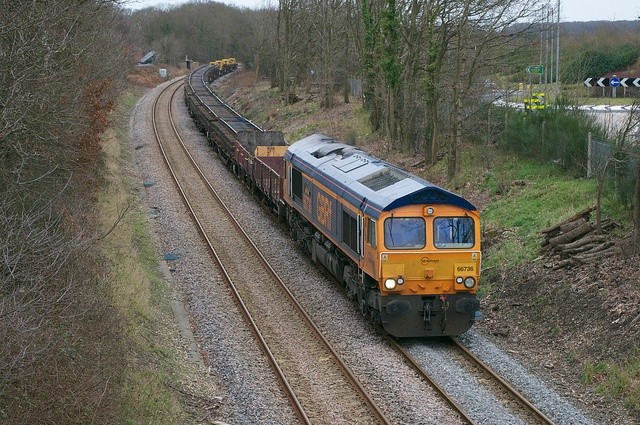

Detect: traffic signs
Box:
528 65 542 74
610 77 620 87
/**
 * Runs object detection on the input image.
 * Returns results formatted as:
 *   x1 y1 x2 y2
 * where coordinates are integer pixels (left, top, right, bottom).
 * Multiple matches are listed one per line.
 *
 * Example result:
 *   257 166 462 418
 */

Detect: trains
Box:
183 57 482 341
